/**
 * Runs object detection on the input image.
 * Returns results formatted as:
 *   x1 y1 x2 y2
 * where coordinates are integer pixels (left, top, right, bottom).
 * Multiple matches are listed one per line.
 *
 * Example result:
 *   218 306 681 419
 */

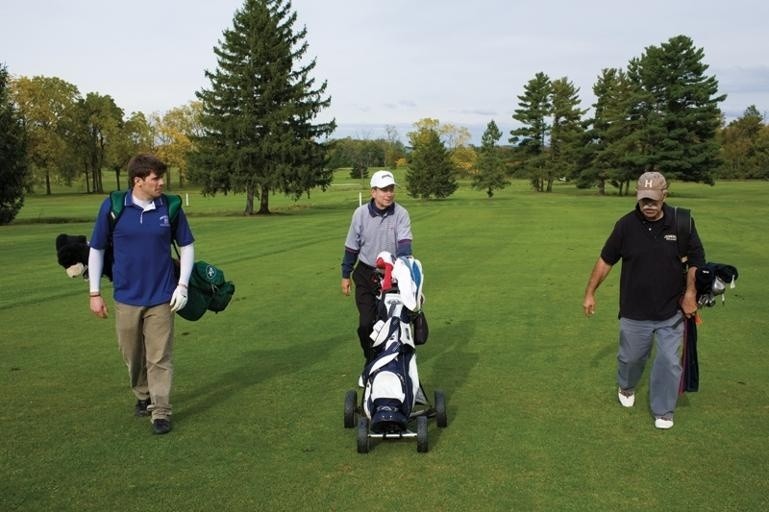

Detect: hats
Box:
636 172 668 202
370 170 402 191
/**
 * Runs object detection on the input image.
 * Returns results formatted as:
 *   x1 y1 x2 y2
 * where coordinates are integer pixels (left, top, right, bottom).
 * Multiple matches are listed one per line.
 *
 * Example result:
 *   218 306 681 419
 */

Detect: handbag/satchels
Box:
173 258 235 321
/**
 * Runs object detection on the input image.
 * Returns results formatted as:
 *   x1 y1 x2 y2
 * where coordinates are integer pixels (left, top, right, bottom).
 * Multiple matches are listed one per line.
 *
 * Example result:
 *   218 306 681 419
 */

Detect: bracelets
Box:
89 294 101 297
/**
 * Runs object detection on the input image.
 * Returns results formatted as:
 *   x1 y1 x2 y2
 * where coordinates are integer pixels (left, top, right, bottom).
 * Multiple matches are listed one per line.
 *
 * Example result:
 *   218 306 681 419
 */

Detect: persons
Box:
341 170 413 387
88 153 196 435
583 172 707 430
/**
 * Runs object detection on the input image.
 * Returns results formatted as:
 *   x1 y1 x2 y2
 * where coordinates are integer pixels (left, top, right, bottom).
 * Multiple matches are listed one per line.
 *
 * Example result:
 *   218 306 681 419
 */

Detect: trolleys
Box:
343 252 448 453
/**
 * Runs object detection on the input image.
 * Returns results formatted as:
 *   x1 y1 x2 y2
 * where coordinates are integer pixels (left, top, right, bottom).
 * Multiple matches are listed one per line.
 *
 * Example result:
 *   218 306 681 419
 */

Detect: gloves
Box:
169 285 188 312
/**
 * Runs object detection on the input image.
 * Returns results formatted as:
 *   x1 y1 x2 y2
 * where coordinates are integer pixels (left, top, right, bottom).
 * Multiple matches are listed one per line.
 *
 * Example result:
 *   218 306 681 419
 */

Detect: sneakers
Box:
358 374 365 388
618 386 635 407
655 414 674 429
136 397 151 417
153 419 170 434
